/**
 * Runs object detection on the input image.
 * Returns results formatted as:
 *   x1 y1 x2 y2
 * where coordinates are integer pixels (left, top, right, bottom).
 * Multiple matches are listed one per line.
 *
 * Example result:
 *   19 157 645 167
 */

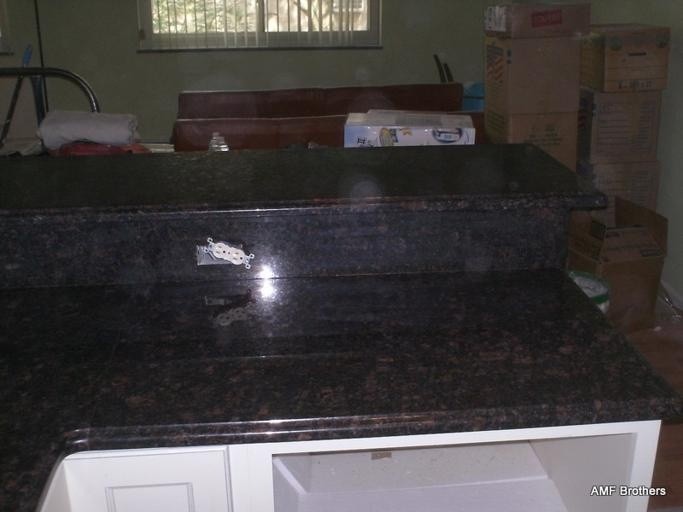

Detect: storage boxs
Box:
343 2 673 337
